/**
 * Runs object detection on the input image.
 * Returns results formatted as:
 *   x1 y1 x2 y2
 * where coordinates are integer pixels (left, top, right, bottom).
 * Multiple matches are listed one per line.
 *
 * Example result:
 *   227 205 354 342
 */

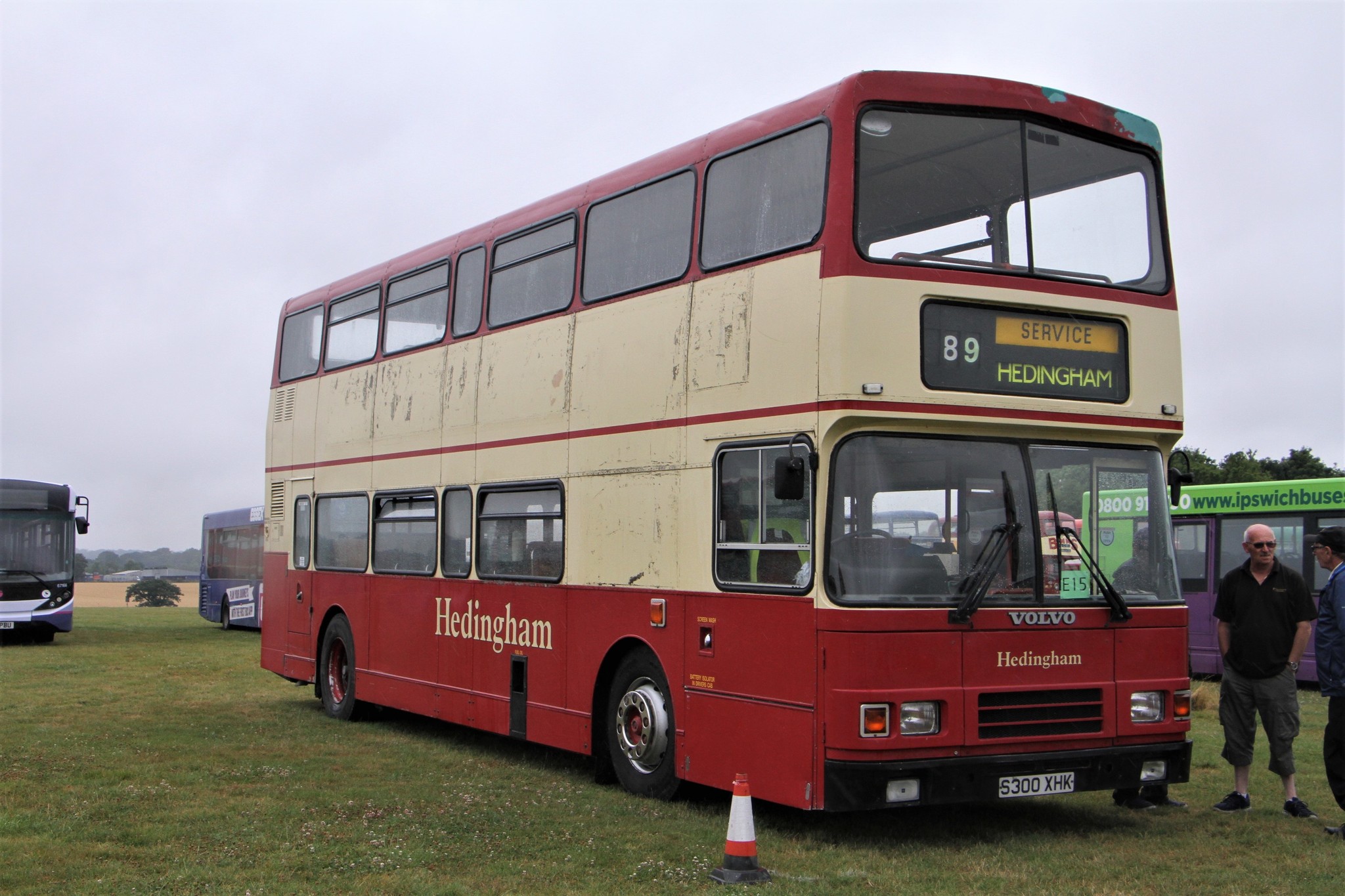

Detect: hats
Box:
1303 526 1345 553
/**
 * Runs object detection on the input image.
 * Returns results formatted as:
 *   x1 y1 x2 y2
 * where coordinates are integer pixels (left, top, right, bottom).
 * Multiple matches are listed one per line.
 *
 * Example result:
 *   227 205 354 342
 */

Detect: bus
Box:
0 475 90 647
258 69 1194 828
1080 474 1345 689
844 511 944 551
929 509 1083 595
198 505 264 630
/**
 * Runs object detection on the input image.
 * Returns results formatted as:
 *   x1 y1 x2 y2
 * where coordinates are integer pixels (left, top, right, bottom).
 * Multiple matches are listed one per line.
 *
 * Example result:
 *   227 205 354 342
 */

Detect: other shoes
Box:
1324 823 1345 840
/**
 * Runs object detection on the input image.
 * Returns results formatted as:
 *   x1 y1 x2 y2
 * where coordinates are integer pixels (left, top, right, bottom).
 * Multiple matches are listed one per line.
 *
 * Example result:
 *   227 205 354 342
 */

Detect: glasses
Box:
1245 541 1277 548
1138 544 1148 551
1311 545 1324 552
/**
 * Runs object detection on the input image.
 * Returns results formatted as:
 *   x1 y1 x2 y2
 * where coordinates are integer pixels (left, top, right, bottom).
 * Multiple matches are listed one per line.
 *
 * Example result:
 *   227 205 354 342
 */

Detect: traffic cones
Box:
707 772 779 887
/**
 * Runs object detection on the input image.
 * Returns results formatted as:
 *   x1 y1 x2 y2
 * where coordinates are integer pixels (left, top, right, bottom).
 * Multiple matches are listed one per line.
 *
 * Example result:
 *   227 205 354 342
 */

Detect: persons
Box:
1310 525 1345 839
1108 526 1187 813
1212 523 1319 821
750 475 810 587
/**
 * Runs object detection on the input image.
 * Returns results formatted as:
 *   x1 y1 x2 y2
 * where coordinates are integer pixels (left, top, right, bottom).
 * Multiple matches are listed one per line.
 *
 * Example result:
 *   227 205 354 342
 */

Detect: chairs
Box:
1175 552 1240 578
524 541 562 577
318 531 452 575
739 519 810 585
1279 552 1302 573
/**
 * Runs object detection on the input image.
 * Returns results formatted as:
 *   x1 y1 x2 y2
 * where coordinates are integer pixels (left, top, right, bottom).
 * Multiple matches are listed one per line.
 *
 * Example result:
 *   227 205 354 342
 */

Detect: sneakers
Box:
1140 793 1188 807
1114 792 1156 810
1213 791 1252 813
1282 797 1319 819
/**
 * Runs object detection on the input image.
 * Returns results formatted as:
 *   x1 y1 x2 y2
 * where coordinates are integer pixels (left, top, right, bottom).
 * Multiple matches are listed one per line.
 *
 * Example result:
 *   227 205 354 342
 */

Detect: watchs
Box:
1286 661 1298 673
